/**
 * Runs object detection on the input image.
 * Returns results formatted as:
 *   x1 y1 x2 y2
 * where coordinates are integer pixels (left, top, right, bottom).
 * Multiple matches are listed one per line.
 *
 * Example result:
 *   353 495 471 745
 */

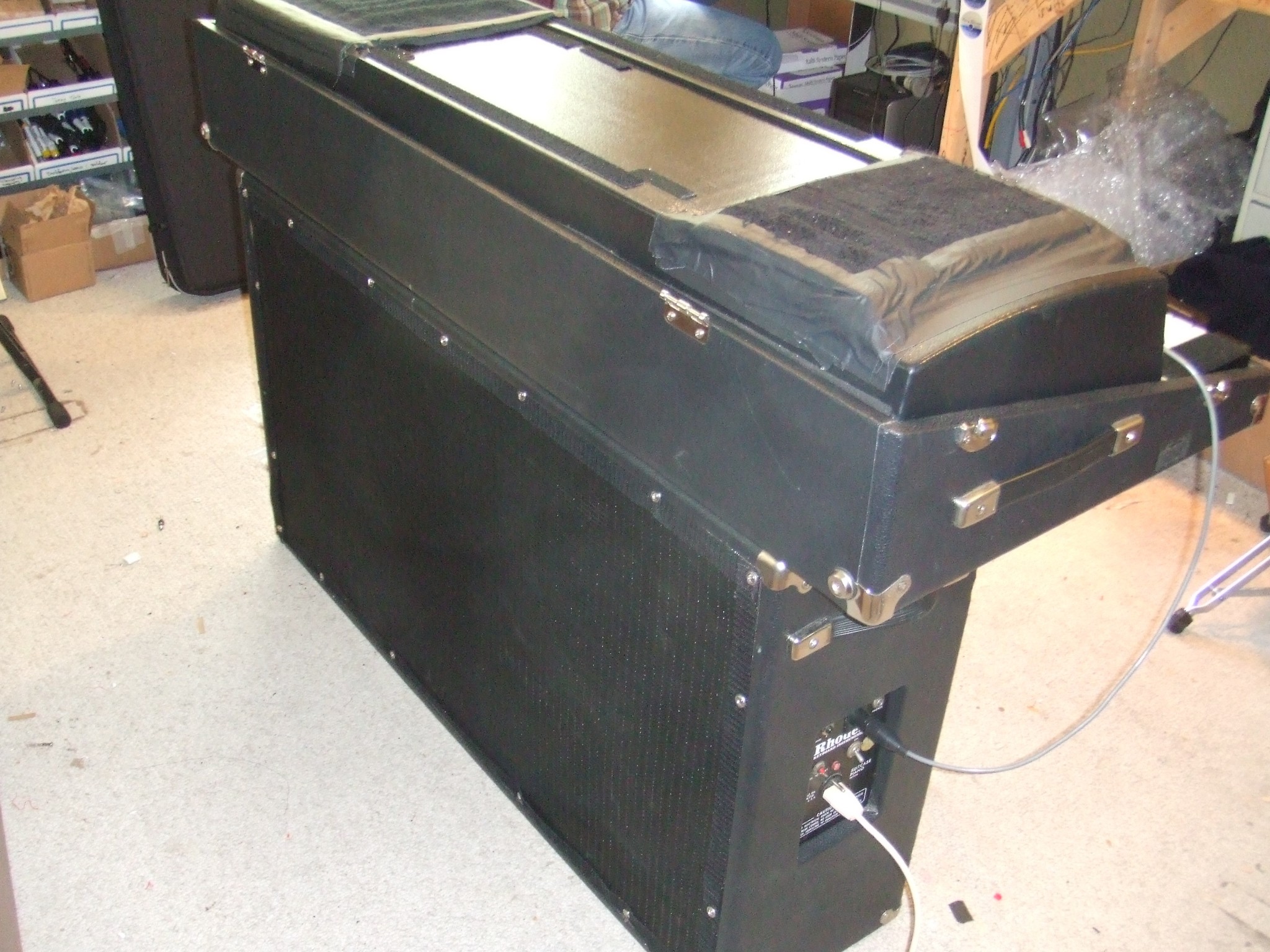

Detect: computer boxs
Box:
825 68 951 156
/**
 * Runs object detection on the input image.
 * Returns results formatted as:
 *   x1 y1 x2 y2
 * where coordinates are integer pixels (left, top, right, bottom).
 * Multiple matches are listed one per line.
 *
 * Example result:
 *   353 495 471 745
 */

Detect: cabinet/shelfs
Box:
707 0 1147 175
0 0 134 199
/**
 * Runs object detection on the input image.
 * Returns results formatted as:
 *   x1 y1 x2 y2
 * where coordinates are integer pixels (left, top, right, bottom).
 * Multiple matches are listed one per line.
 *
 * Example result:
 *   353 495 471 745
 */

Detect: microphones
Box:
892 73 933 99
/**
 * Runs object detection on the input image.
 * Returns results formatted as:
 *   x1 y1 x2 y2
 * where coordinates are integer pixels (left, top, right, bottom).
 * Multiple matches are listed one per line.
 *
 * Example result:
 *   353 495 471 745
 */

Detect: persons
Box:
551 0 782 90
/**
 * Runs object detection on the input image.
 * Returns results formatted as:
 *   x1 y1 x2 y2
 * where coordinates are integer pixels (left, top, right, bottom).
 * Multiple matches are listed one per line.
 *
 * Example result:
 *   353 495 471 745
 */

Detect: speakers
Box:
234 172 972 950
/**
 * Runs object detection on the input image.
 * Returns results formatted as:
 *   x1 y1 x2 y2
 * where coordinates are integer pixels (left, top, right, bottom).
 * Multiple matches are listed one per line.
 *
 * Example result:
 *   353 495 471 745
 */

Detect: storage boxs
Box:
0 0 156 302
758 25 851 116
